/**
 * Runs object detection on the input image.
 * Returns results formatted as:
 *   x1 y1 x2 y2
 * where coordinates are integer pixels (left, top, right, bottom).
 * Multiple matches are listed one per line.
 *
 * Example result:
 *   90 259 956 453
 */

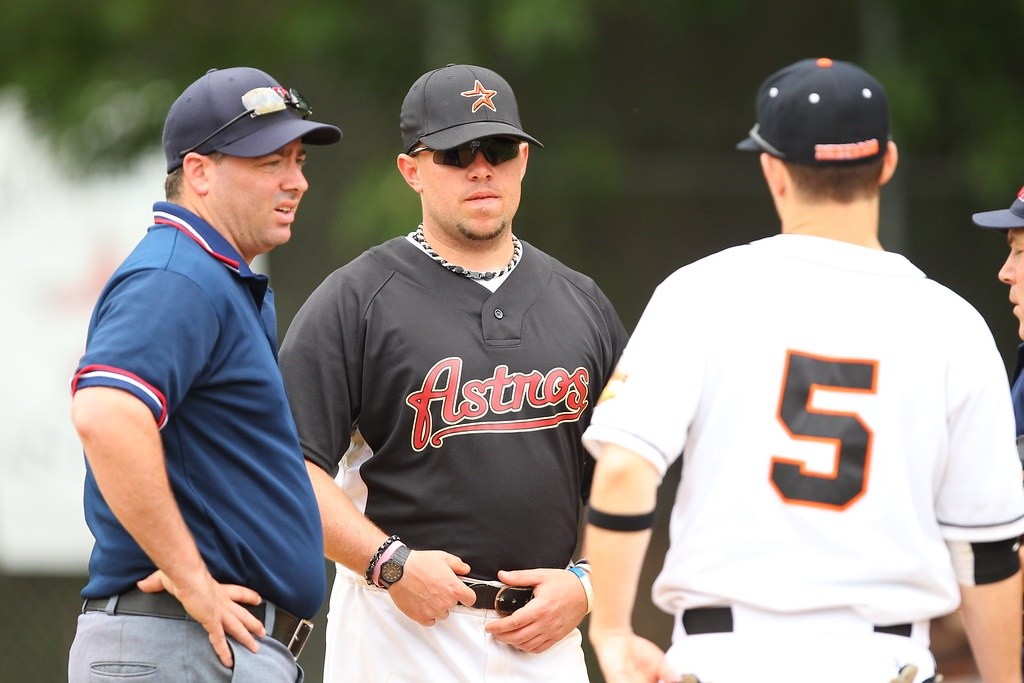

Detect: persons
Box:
67 70 341 683
278 62 630 683
581 58 1024 683
971 184 1024 486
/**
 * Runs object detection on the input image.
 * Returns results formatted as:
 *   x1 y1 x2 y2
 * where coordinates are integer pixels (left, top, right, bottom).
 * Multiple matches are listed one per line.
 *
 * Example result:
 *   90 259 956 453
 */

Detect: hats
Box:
736 58 890 166
400 63 544 154
972 185 1024 234
162 66 343 174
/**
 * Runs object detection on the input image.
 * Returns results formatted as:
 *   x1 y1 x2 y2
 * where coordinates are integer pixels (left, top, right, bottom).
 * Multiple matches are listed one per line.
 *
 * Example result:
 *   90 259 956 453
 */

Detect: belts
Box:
457 581 535 618
85 585 314 661
683 607 912 637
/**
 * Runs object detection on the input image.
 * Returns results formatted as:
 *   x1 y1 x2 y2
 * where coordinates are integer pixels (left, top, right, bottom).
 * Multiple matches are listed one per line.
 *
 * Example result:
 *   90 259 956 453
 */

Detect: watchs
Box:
378 546 411 590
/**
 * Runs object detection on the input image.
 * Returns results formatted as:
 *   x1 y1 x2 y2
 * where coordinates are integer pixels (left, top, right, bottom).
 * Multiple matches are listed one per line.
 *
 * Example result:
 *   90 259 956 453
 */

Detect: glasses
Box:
179 88 313 158
409 136 526 168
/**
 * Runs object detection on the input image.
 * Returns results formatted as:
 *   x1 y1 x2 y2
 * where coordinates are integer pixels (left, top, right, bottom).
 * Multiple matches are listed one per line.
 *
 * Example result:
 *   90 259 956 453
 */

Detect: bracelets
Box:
568 558 595 616
372 541 407 588
365 534 400 589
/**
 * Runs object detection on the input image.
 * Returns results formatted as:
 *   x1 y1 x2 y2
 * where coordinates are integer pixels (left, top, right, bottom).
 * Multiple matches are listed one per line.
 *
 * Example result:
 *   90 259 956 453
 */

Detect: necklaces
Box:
415 222 521 280
